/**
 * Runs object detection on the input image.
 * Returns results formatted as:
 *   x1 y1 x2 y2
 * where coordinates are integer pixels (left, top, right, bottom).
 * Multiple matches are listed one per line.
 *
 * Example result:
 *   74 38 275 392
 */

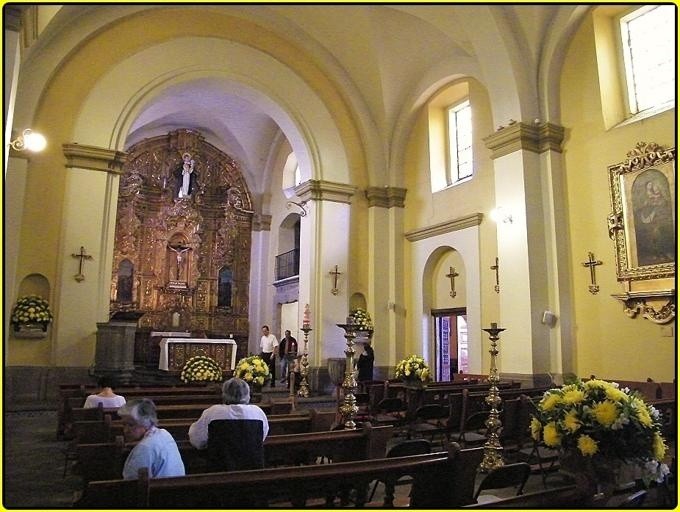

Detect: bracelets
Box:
271 352 274 357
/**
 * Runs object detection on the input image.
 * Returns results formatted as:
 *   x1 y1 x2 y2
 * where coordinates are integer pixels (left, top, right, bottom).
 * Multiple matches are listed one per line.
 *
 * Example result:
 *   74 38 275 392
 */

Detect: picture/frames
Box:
607 139 677 283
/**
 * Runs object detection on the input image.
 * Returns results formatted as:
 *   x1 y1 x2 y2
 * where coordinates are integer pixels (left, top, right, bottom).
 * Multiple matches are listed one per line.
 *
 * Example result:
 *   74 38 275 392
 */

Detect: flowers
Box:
10 294 53 325
233 352 273 386
394 353 433 382
349 308 374 330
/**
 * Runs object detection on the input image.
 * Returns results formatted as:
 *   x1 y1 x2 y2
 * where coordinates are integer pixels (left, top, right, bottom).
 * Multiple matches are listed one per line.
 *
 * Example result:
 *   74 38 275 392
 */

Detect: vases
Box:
19 322 44 331
353 330 370 337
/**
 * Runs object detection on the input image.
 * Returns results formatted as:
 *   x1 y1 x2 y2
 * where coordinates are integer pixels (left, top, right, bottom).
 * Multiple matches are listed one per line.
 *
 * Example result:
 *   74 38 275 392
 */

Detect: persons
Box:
278 330 297 384
355 342 374 380
168 246 189 280
175 151 193 197
83 375 126 409
259 325 279 387
188 378 270 450
640 180 666 260
117 397 187 480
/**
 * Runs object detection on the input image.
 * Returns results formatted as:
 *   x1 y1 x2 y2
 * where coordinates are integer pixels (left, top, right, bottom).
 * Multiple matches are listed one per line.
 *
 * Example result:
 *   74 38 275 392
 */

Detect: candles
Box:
346 316 354 325
291 343 295 351
491 322 498 329
